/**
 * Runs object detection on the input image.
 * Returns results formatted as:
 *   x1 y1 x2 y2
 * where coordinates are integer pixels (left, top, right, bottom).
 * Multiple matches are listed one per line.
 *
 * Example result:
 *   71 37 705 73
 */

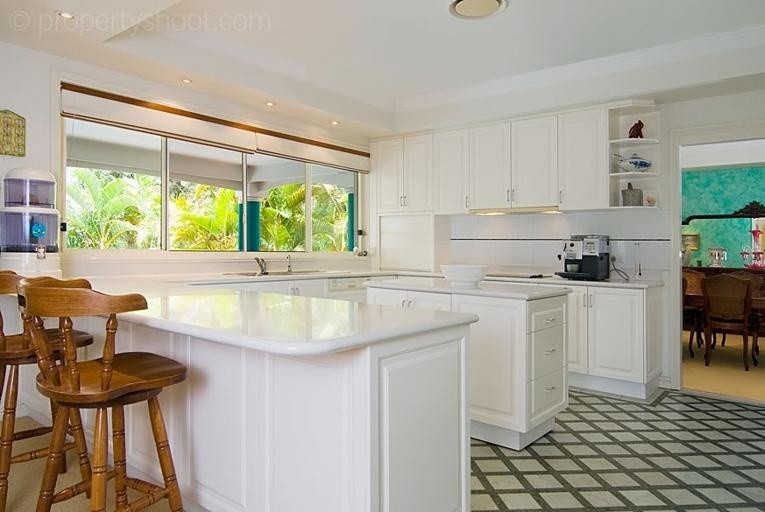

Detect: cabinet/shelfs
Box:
527 294 569 432
257 279 324 298
452 295 527 435
369 133 432 213
367 286 452 311
608 104 663 209
538 284 664 385
558 107 610 211
469 113 559 210
432 128 469 215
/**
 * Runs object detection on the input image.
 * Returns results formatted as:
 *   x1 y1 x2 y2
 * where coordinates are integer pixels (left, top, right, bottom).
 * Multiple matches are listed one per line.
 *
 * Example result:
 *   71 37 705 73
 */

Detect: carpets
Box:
470 386 765 511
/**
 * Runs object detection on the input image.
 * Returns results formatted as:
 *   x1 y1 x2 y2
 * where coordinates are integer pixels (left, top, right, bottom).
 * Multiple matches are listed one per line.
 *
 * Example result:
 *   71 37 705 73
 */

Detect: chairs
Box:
15 275 187 512
682 266 765 371
1 269 93 512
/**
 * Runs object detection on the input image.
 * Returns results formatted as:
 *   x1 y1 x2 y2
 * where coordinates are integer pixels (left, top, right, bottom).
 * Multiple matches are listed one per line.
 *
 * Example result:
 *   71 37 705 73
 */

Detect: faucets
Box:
254 257 266 273
286 255 293 270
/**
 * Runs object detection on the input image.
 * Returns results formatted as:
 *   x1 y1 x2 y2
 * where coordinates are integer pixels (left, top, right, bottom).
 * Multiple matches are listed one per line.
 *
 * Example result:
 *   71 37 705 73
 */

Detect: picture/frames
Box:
681 233 700 251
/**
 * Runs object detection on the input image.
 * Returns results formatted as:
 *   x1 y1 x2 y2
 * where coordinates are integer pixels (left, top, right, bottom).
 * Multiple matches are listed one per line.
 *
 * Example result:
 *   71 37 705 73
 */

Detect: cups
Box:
697 257 703 268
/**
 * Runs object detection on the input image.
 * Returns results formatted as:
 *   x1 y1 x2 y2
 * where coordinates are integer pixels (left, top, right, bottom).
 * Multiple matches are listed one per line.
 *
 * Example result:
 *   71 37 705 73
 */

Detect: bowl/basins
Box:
440 263 489 289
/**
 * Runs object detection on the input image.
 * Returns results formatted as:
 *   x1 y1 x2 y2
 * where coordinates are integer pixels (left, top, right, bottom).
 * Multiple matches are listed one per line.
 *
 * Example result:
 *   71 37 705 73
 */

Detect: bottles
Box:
620 183 644 207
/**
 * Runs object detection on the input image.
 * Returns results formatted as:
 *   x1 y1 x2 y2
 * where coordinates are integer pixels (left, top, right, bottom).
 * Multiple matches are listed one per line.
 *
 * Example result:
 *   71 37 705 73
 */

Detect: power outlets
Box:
611 250 624 263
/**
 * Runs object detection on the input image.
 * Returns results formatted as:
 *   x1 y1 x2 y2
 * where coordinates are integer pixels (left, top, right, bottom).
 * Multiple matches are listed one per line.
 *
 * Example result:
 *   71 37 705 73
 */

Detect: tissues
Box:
622 183 643 205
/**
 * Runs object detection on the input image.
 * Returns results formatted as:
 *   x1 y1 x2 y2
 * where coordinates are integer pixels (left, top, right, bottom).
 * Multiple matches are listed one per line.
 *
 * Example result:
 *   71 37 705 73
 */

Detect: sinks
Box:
223 272 291 276
290 270 324 274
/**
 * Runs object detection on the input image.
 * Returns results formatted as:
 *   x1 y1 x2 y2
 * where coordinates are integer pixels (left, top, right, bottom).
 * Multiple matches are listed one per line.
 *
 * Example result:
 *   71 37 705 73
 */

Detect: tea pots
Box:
612 151 652 171
707 248 728 269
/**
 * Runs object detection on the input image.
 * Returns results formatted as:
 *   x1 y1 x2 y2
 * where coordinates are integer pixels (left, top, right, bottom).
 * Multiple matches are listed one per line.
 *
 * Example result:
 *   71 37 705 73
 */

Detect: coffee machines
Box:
554 233 610 283
